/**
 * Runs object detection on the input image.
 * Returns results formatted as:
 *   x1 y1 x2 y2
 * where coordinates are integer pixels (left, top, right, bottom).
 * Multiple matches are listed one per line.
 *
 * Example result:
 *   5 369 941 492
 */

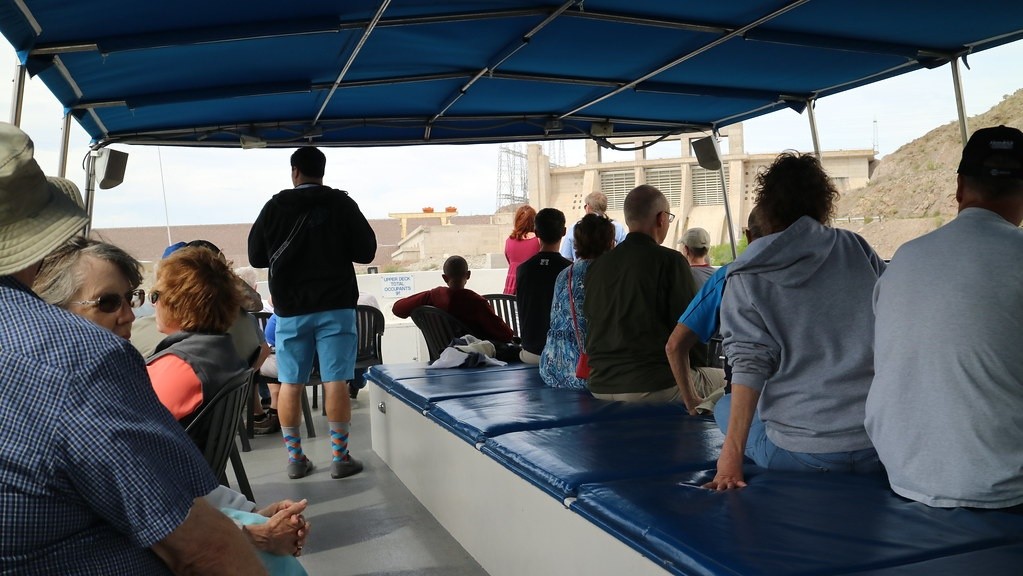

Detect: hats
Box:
957 125 1023 181
678 228 711 248
162 242 187 260
0 122 92 276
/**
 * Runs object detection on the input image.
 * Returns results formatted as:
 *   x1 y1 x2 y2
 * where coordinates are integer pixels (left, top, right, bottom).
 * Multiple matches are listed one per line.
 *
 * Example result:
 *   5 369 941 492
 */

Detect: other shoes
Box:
348 381 359 398
261 397 271 409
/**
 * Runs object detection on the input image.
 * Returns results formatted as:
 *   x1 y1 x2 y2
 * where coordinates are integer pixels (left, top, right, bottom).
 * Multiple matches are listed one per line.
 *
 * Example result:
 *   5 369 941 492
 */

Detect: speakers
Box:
691 135 723 171
94 148 129 189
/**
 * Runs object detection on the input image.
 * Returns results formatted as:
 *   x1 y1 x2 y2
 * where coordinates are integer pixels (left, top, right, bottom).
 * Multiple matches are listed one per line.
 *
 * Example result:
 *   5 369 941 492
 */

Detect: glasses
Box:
148 291 159 303
70 289 145 313
657 211 675 222
584 204 587 209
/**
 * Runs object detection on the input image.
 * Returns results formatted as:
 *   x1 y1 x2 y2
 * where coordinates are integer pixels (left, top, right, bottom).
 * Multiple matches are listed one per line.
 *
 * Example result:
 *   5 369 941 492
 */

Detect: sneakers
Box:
331 450 363 478
237 416 281 434
287 455 313 479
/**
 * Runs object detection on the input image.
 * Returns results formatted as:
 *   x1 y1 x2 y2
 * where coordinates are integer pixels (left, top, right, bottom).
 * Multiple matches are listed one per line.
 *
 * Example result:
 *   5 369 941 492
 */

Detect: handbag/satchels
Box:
576 354 593 380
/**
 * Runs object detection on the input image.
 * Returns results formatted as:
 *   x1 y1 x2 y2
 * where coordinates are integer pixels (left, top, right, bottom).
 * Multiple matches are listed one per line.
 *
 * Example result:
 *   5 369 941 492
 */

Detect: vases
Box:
422 207 434 213
446 207 456 212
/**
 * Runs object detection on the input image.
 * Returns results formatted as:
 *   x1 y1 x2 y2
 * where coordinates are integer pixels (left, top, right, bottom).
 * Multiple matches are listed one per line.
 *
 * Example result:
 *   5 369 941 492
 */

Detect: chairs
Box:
480 293 520 343
184 367 257 511
247 304 389 438
410 304 472 363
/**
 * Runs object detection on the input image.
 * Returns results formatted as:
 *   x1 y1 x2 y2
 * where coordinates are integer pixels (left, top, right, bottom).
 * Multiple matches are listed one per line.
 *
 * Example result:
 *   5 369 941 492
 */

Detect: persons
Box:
504 184 763 417
392 256 522 344
861 123 1023 515
33 235 312 576
0 120 271 576
699 149 886 492
248 146 378 480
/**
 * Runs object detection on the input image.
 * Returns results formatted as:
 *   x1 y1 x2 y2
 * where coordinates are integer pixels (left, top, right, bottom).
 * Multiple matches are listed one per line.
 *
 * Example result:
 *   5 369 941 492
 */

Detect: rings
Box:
297 541 303 550
